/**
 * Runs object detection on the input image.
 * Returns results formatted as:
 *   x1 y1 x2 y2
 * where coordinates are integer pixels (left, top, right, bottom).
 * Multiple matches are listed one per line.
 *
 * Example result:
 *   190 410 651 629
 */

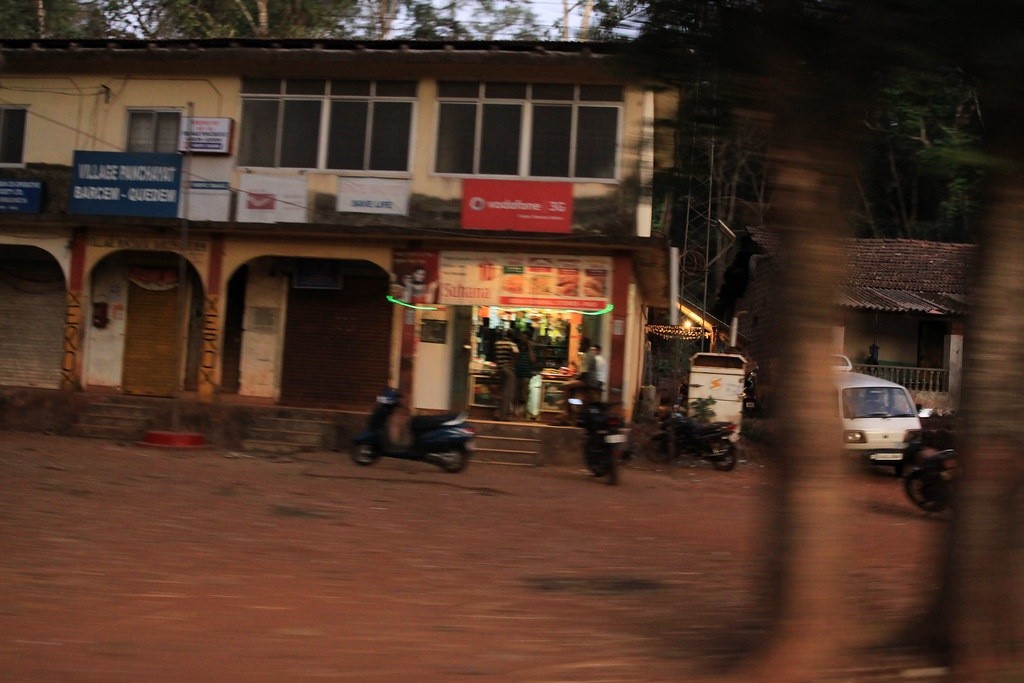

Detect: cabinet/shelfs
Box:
540 379 567 412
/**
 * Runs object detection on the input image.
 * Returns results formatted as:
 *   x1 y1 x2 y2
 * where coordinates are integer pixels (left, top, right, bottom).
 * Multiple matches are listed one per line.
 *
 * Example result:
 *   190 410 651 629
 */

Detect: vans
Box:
833 356 930 478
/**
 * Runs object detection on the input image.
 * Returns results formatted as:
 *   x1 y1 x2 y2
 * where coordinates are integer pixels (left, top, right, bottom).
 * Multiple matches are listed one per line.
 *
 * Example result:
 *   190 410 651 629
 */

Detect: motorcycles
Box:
567 397 633 486
740 371 760 421
905 441 966 523
645 406 738 471
686 353 748 441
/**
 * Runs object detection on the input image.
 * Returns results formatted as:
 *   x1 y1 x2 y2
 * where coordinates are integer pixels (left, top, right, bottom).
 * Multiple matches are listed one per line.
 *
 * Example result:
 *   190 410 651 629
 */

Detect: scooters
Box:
350 380 477 473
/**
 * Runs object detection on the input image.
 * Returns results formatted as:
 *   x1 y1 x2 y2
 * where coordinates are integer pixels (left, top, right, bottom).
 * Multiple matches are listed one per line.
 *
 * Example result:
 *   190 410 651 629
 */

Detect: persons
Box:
514 330 536 406
401 267 437 304
494 329 522 421
590 343 607 391
555 337 597 422
